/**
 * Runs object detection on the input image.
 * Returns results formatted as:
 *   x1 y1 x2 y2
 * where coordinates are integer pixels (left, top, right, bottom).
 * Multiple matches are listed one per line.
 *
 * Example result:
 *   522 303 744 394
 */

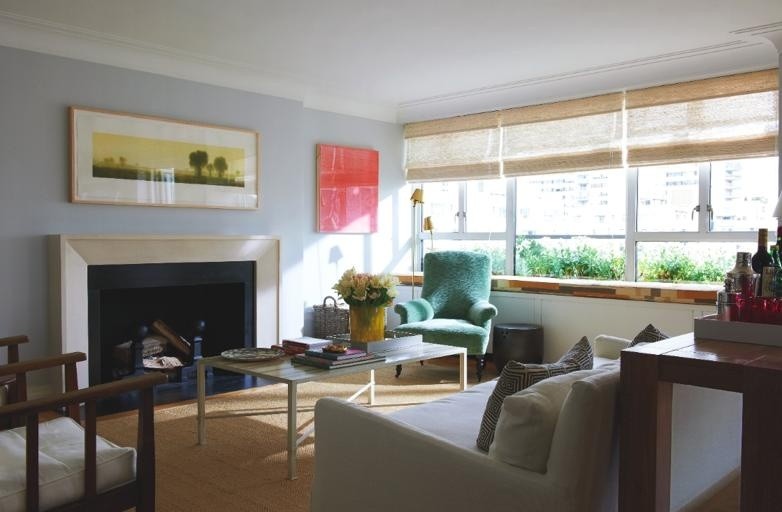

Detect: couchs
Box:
310 334 743 512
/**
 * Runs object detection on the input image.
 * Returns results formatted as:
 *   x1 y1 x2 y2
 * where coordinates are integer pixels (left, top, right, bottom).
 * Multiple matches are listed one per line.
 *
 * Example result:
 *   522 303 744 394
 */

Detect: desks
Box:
620 330 782 512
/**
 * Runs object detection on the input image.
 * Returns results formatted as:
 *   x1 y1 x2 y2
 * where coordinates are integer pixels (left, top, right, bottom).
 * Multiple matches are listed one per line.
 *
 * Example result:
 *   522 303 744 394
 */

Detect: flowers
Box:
331 266 401 330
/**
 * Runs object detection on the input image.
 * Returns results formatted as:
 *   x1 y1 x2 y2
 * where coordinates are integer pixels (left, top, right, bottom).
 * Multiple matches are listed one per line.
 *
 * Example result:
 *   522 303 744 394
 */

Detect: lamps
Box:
410 188 425 301
424 215 435 252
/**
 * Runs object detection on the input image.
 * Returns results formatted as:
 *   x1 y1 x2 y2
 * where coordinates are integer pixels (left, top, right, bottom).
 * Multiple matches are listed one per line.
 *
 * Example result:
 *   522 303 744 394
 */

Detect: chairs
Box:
393 250 498 382
0 335 168 512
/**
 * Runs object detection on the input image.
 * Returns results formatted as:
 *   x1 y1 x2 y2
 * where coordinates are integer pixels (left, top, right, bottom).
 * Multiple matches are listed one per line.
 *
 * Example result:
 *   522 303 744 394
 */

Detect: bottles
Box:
718 224 782 322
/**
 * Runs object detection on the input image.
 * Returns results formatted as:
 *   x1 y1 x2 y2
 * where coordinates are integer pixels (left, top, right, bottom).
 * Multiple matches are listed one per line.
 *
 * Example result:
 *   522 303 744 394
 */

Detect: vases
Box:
349 304 386 346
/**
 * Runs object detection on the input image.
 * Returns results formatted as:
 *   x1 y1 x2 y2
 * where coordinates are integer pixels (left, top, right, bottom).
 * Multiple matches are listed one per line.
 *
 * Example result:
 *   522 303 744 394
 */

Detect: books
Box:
282 336 387 370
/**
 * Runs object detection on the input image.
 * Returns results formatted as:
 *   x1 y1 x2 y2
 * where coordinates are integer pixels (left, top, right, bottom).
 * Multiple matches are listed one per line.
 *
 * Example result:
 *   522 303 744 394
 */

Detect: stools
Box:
492 322 545 373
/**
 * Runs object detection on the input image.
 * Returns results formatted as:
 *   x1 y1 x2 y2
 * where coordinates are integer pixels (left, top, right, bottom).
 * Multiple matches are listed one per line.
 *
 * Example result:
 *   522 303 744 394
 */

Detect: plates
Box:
221 346 285 361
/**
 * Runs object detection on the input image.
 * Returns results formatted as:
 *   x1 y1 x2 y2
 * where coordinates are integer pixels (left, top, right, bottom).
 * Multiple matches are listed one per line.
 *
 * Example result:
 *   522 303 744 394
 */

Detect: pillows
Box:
487 364 620 473
476 334 594 451
617 324 670 358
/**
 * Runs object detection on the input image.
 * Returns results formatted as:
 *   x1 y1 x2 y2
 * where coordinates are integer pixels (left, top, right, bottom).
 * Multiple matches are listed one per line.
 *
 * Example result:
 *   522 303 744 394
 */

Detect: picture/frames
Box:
69 103 261 210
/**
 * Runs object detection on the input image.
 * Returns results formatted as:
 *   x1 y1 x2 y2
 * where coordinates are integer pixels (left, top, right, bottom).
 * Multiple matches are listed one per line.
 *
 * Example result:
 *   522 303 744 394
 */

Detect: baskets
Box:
312 295 352 338
